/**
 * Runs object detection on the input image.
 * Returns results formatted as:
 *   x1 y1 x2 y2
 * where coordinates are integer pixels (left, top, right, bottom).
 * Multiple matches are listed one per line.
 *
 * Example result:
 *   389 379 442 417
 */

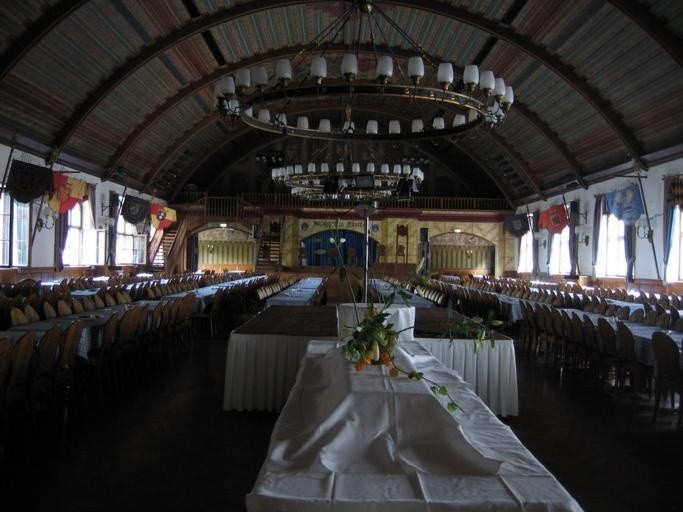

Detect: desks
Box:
247 335 578 512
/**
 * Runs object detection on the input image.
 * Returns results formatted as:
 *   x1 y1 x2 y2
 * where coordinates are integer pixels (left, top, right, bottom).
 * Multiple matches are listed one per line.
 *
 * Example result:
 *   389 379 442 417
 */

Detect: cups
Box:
291 286 297 297
298 282 304 290
